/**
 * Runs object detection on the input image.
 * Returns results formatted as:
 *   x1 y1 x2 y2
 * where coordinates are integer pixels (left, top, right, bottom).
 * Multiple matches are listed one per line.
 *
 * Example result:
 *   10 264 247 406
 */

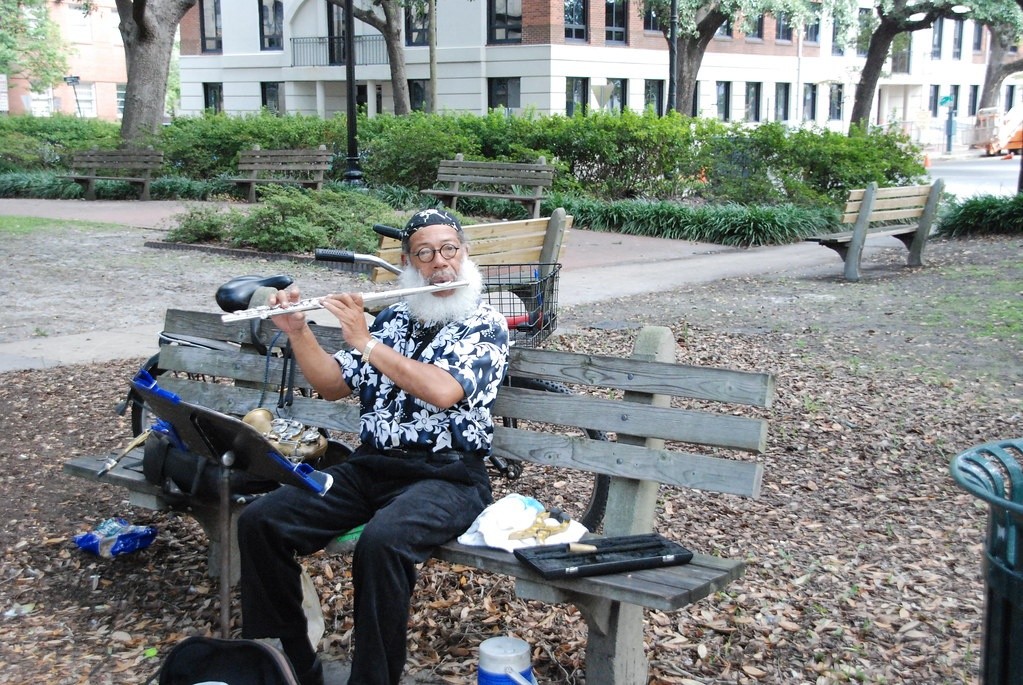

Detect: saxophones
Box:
97 409 328 477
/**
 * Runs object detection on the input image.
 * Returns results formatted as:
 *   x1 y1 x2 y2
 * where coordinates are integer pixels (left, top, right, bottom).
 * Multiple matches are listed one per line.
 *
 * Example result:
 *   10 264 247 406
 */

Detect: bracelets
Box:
361 339 378 362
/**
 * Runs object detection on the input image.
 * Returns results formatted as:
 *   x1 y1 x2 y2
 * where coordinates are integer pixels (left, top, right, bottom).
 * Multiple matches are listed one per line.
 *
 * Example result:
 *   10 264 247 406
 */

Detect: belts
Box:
365 442 480 462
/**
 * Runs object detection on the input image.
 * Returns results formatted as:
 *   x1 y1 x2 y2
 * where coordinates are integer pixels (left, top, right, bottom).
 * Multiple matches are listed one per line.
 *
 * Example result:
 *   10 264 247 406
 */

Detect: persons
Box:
238 209 508 684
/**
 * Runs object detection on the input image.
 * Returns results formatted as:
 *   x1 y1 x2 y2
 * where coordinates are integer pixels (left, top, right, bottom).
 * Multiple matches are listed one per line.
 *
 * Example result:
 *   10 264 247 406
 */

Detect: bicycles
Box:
116 225 611 532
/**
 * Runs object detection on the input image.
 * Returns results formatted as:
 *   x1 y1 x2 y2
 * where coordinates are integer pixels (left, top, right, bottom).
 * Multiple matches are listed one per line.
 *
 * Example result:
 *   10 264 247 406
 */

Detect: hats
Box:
401 208 464 249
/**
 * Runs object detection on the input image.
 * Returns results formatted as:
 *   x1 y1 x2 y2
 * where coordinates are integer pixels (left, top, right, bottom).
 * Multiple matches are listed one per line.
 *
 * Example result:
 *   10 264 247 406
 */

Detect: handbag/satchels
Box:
158 636 299 685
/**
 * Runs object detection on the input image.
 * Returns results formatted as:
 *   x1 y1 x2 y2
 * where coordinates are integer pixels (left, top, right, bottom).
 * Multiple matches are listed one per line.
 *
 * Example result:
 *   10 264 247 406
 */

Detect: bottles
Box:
478 636 538 685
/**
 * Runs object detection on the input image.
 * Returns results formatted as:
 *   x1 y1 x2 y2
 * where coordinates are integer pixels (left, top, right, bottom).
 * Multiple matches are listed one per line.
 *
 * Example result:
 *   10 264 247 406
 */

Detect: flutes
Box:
221 281 470 325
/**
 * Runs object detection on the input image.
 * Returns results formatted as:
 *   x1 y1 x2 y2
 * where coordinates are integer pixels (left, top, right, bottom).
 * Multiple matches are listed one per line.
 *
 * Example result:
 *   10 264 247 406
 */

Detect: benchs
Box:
56 144 164 202
364 207 574 336
225 145 333 204
806 177 944 280
64 286 776 685
419 153 554 219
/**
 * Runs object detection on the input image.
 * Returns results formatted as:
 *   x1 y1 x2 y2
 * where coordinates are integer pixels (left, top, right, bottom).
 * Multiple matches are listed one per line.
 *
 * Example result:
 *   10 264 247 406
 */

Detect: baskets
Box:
476 262 562 348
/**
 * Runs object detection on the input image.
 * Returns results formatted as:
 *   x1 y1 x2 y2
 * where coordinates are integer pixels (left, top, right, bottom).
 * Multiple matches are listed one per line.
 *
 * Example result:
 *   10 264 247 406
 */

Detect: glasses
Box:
409 242 464 263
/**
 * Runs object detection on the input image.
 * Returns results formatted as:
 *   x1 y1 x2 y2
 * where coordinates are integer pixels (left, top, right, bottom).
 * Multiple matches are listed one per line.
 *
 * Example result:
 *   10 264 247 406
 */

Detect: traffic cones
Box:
1000 155 1012 160
924 154 933 169
